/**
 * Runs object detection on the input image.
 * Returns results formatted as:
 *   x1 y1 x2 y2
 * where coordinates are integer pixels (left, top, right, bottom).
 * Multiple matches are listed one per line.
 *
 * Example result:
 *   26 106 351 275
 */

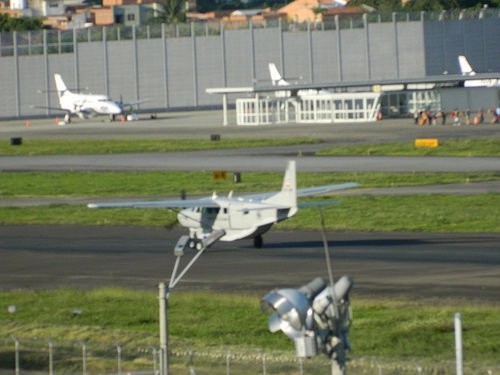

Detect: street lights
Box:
260 274 352 375
156 229 227 375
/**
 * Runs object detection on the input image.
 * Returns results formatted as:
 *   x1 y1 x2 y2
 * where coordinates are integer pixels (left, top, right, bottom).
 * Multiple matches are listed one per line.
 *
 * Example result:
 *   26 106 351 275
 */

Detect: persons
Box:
406 105 500 128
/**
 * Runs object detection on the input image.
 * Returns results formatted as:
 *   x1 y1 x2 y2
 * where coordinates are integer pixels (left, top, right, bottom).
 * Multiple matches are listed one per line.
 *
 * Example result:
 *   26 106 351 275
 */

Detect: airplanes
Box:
254 63 334 109
30 73 153 123
458 55 500 90
87 160 358 252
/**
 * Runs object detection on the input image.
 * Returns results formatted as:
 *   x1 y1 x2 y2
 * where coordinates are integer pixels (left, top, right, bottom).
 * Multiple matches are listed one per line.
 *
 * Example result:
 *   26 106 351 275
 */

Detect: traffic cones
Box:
24 114 128 126
